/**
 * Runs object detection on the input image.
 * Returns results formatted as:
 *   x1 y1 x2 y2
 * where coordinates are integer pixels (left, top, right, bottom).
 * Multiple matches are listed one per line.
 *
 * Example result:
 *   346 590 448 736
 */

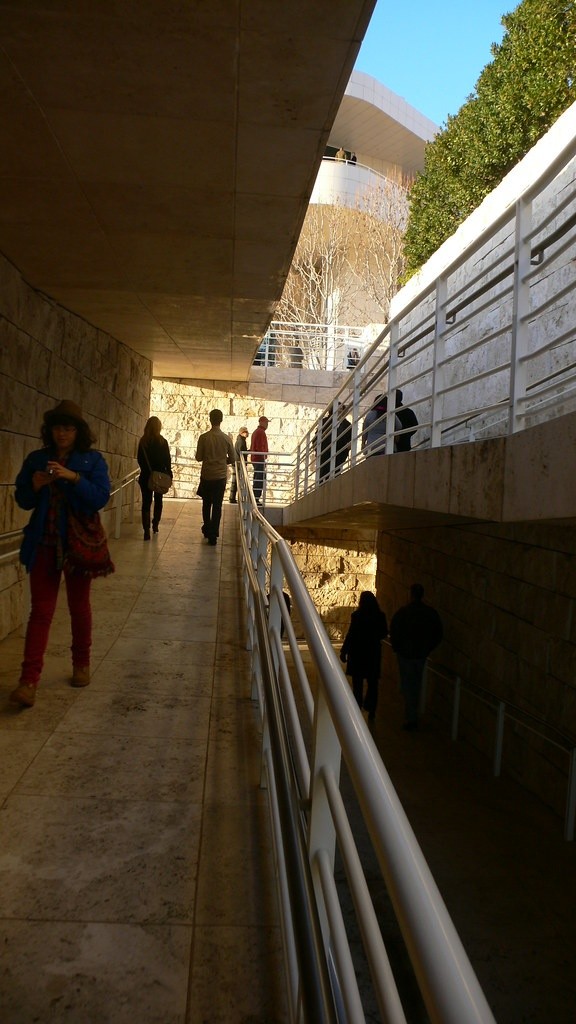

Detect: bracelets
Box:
74 473 80 482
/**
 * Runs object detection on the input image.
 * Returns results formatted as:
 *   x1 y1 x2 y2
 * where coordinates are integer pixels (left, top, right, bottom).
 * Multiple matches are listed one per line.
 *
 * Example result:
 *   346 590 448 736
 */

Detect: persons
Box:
135 415 173 541
225 425 252 502
265 591 292 639
194 408 236 546
388 387 418 453
251 330 277 366
345 347 361 368
390 580 444 724
362 392 402 459
339 590 389 720
312 400 355 483
3 397 113 707
286 337 305 368
251 416 271 505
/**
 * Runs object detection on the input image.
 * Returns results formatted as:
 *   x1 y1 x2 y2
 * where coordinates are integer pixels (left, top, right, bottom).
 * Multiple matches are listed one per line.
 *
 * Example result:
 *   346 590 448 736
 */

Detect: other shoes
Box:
145 531 150 540
11 683 38 706
152 525 159 532
209 536 217 545
204 532 210 537
72 663 91 684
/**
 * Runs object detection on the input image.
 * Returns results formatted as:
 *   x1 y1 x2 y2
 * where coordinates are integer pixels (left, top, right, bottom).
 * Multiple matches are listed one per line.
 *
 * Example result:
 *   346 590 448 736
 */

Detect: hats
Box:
260 416 271 422
239 427 247 434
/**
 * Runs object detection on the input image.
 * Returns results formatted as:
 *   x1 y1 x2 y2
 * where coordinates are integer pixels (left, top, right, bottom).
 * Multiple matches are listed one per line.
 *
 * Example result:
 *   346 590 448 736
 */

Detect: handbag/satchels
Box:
51 501 114 577
149 471 171 492
